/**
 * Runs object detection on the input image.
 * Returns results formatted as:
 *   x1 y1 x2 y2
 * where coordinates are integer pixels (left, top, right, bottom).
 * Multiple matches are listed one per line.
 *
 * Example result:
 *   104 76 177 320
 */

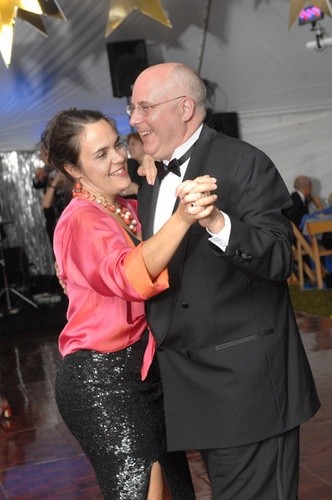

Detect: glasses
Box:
126 96 187 116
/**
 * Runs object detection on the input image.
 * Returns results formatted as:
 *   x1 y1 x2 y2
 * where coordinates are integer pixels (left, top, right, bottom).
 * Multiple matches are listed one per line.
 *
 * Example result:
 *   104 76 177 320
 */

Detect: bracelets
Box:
49 184 57 189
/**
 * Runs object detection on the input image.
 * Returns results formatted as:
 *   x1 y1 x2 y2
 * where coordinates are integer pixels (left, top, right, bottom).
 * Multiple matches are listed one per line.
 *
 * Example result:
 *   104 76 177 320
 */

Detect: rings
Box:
190 203 195 208
204 192 207 198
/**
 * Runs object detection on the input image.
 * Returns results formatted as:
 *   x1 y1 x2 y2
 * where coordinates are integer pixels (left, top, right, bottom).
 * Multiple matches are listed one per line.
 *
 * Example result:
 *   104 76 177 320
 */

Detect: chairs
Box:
286 209 332 290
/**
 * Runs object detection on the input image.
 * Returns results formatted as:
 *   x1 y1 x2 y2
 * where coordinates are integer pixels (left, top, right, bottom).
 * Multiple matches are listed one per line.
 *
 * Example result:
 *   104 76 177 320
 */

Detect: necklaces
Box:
72 186 137 236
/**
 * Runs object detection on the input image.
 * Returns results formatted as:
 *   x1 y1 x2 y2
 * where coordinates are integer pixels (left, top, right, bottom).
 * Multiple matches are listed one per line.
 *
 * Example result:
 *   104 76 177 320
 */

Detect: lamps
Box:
298 5 323 48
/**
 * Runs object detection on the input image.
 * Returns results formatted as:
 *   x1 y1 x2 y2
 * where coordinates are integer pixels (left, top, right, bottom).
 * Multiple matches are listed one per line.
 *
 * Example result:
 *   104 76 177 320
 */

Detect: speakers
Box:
105 40 149 98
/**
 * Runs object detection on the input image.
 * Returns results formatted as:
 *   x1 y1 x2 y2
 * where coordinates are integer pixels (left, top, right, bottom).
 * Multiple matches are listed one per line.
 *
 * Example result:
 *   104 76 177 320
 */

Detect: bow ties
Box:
154 141 198 179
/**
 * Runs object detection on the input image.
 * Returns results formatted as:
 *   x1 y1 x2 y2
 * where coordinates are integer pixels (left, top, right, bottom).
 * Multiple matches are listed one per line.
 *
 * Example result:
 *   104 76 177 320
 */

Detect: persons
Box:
125 132 146 185
46 107 218 500
281 175 323 227
125 63 321 500
32 145 70 325
